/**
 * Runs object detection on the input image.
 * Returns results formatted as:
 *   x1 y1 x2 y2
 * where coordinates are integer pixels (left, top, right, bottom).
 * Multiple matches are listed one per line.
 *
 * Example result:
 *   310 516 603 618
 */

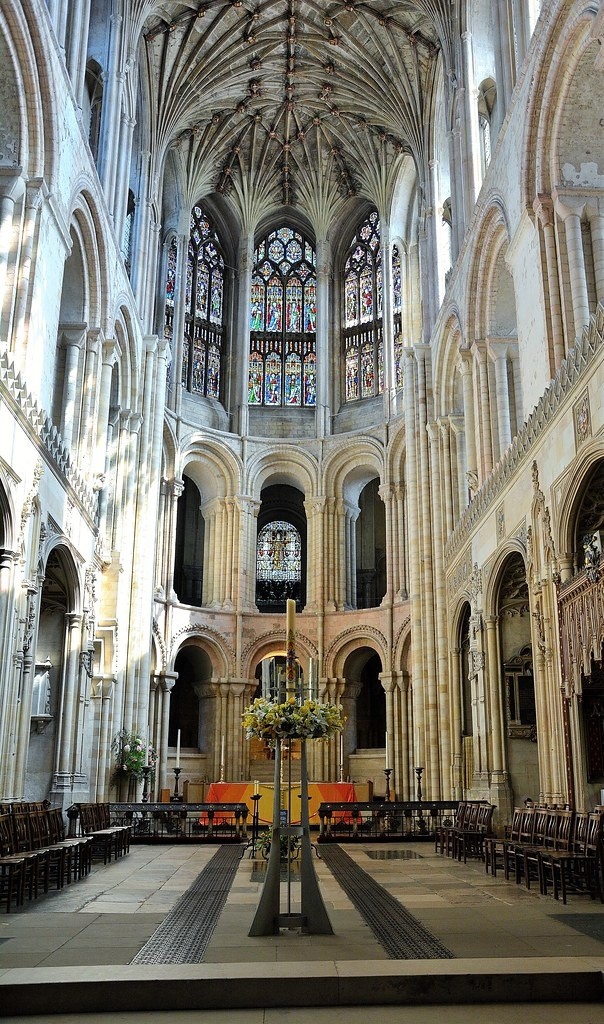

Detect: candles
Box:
341 735 343 764
145 726 148 766
386 732 389 769
417 727 420 767
176 729 180 768
221 735 225 764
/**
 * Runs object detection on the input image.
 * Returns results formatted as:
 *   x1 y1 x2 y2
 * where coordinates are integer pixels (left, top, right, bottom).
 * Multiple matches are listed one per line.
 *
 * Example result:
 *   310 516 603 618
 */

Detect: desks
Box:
198 782 363 826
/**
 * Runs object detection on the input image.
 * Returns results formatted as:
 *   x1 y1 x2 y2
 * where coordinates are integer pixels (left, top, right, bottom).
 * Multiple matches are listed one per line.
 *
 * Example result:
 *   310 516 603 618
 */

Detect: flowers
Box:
108 728 158 791
240 697 345 741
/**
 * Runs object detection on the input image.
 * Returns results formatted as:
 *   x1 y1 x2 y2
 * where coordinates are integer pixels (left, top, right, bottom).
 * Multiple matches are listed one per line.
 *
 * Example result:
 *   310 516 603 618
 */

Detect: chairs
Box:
0 799 132 913
434 798 604 904
